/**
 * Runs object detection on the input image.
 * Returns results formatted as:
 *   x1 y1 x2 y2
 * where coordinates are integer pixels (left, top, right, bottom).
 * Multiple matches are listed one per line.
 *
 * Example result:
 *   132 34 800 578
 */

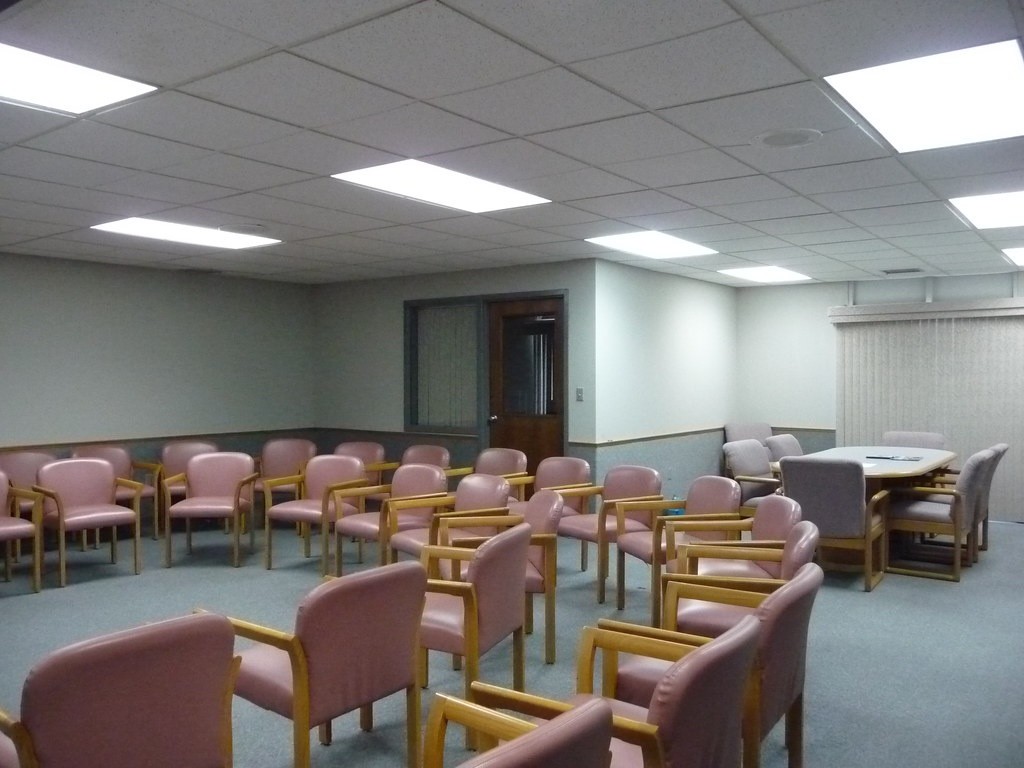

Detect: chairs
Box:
887 449 996 583
884 431 946 538
0 425 891 768
907 441 1010 564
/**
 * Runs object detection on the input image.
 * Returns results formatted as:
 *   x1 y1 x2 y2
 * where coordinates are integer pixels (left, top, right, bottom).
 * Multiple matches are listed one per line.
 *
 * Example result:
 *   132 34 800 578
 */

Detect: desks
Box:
772 446 958 567
